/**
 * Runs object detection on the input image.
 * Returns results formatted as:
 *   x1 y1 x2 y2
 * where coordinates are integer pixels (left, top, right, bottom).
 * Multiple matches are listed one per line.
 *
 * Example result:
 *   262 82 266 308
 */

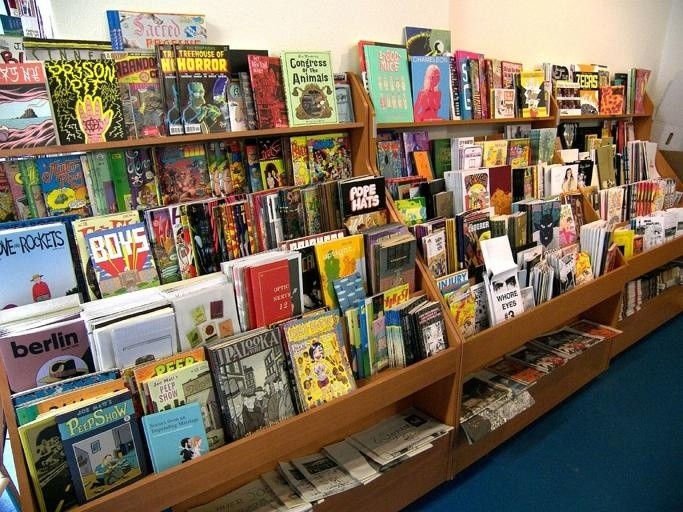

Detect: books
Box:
2 0 683 511
